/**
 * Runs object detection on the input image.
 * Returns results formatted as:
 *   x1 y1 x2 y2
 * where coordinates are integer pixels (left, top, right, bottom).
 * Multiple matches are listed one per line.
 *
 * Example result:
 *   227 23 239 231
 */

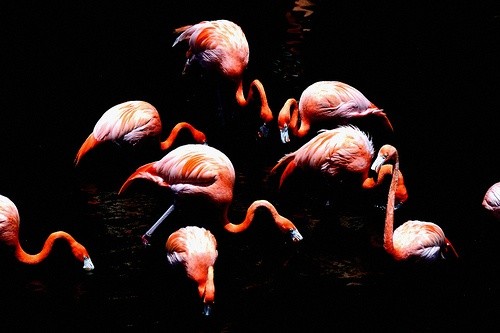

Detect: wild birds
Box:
369 144 460 262
270 124 408 211
482 182 500 211
0 195 95 271
172 20 274 139
73 101 207 165
278 81 393 143
166 225 218 316
117 143 305 246
